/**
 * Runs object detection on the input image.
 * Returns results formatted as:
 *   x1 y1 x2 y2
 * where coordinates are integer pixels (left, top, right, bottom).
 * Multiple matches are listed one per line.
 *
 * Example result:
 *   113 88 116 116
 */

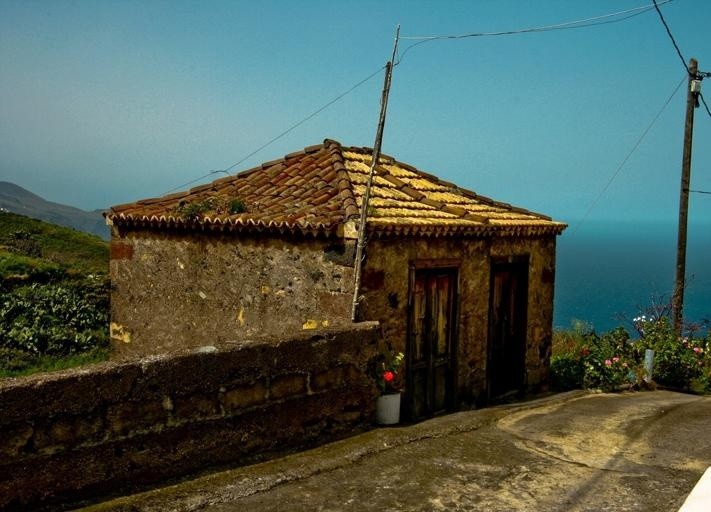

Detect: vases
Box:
377 395 401 425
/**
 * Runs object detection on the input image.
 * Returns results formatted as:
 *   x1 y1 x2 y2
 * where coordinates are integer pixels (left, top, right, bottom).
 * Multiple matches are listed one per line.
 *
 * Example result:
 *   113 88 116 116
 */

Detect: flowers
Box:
372 352 405 394
